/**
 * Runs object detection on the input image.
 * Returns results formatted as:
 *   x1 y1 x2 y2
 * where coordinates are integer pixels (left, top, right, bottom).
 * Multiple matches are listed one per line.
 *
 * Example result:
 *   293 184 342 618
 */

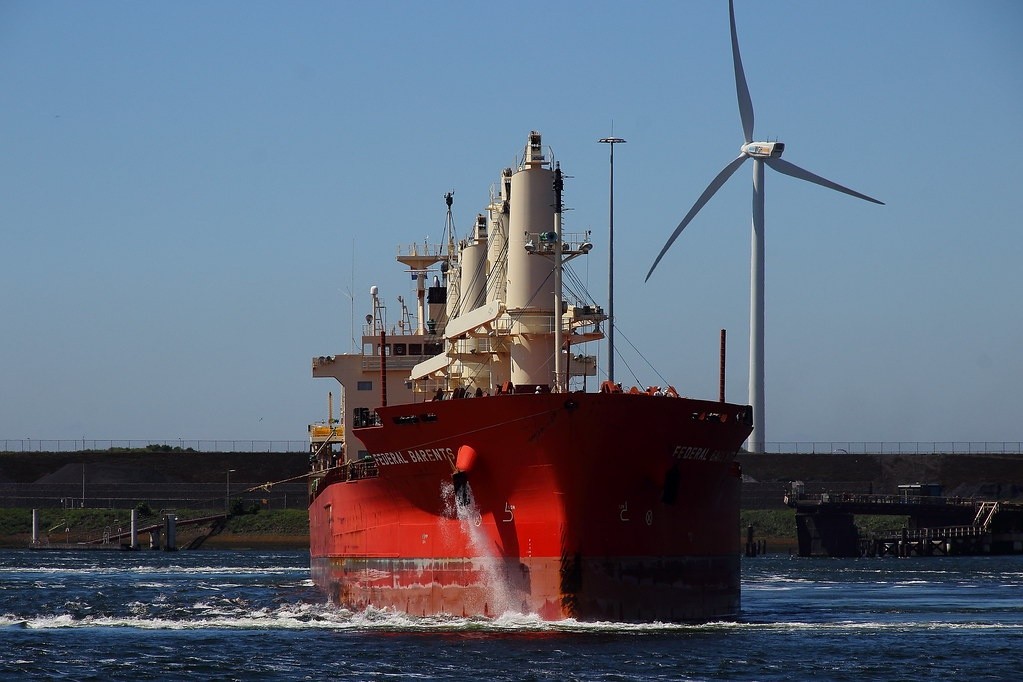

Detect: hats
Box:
618 382 622 385
657 386 661 389
535 386 541 390
646 386 649 390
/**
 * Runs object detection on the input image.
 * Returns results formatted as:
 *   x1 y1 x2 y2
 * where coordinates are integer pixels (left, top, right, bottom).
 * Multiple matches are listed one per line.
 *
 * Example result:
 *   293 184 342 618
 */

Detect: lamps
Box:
578 241 593 252
525 239 536 253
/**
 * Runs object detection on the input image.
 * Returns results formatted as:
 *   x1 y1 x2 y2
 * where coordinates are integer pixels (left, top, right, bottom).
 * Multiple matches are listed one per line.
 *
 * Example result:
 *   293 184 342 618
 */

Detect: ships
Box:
305 128 755 633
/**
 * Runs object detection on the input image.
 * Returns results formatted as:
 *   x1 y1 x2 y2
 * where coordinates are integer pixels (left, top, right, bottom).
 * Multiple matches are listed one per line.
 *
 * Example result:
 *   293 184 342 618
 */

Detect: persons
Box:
347 457 354 480
615 382 663 396
432 383 502 401
535 385 559 393
427 318 437 334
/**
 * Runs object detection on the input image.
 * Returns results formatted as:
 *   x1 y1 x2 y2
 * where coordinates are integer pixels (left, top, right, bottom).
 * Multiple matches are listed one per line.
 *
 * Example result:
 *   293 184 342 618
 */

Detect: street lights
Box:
227 470 236 511
596 116 628 385
